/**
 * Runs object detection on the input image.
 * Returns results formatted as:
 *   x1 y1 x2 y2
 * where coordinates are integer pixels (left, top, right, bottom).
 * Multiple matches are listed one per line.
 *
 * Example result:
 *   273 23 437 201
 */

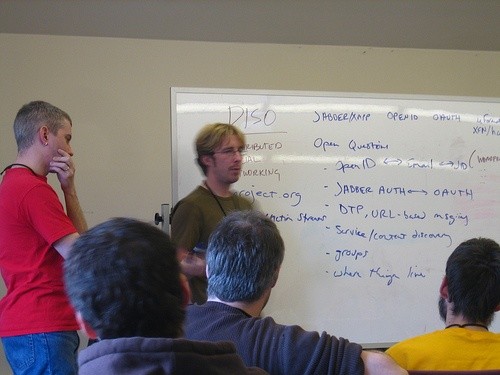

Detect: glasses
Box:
215 148 249 156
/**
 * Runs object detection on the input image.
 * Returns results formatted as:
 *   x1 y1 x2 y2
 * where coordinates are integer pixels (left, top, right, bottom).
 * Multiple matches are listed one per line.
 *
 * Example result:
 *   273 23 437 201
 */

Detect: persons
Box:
382 237 500 375
168 121 262 302
180 211 409 375
63 215 270 375
0 100 90 375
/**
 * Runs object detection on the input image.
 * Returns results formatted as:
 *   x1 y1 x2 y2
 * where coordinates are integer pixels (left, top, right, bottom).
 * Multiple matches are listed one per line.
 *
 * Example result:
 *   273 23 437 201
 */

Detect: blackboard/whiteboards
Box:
170 86 500 349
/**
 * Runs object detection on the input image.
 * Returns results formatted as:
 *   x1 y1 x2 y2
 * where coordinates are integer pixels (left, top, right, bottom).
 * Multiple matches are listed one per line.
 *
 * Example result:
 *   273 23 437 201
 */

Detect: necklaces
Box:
204 179 229 219
444 322 491 331
0 163 38 178
